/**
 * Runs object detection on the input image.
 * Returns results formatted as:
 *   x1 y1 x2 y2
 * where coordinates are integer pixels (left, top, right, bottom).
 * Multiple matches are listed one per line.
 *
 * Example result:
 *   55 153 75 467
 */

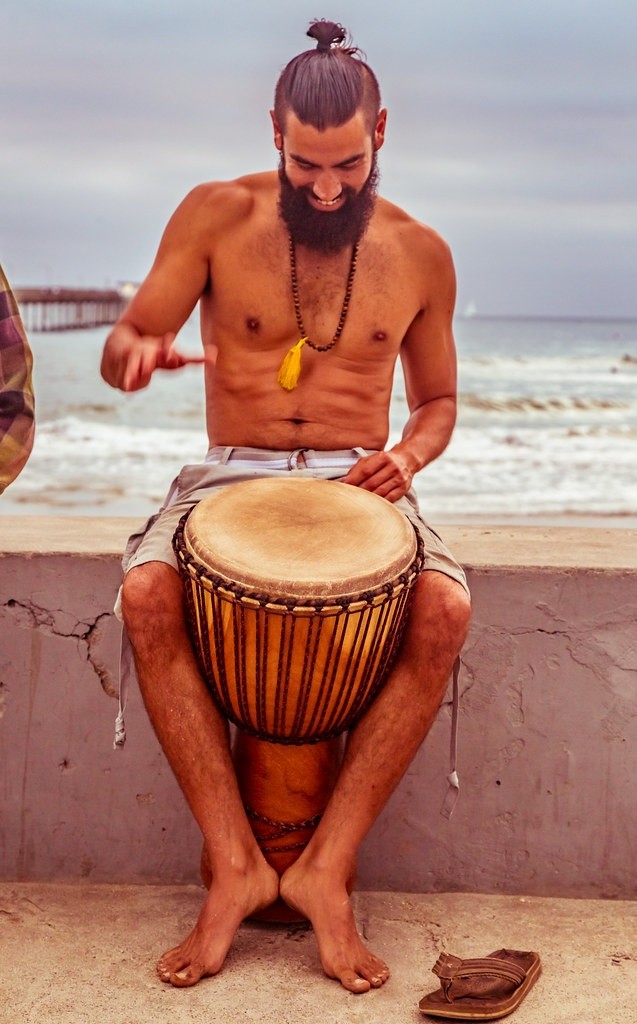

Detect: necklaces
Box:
278 229 362 391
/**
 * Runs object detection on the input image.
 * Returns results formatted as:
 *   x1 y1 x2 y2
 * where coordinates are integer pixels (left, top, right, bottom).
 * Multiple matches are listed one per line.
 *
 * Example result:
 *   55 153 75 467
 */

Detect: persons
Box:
0 265 35 495
98 21 475 993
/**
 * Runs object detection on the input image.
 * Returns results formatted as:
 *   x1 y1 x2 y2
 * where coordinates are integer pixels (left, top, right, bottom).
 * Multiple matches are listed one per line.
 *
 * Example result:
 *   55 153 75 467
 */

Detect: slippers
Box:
419 948 542 1019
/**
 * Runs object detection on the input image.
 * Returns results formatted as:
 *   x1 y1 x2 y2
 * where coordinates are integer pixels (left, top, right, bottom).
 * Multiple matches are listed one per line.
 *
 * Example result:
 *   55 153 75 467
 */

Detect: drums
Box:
171 474 427 925
